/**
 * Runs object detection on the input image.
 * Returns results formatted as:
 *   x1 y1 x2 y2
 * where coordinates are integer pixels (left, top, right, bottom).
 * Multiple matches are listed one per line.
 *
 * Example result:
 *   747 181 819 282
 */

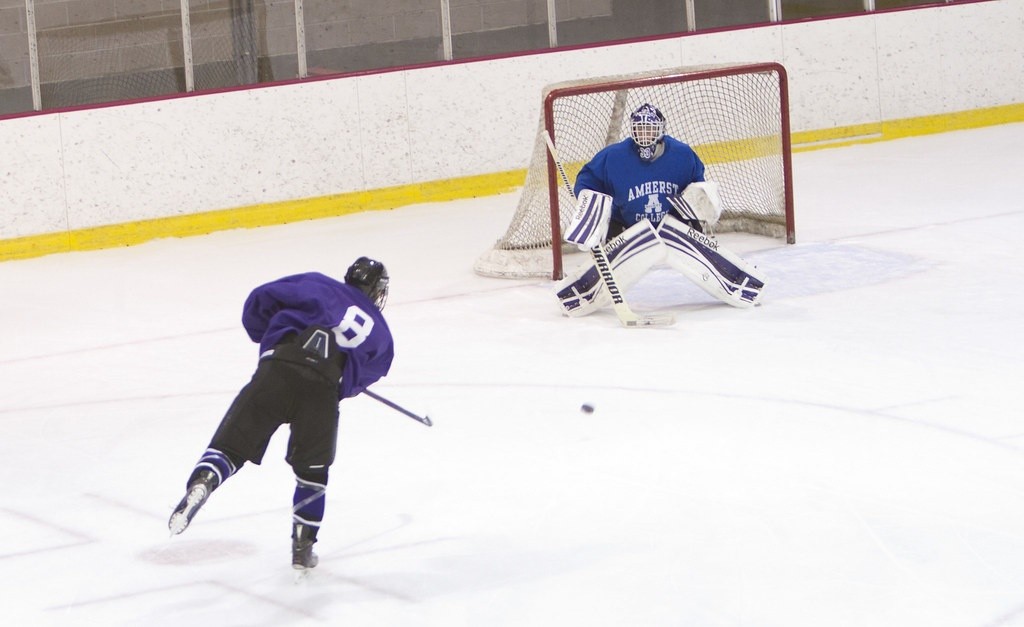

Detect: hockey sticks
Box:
541 130 675 327
362 388 433 427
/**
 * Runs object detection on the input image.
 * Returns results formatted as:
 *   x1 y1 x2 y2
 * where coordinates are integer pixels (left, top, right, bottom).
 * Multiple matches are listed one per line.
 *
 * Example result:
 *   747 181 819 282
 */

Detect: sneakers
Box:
169 470 219 534
291 523 319 570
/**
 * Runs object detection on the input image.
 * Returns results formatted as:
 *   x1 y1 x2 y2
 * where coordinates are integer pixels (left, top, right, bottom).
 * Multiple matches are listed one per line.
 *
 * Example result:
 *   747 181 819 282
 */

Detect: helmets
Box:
344 257 390 309
630 103 666 161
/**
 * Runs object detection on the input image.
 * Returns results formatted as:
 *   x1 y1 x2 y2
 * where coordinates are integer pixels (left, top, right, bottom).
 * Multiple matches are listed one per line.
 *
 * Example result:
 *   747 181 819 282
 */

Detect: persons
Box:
168 256 394 584
556 103 764 317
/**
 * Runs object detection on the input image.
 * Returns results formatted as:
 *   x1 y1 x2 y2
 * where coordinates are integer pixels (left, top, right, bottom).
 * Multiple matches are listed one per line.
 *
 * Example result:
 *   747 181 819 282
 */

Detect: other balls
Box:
580 404 594 414
